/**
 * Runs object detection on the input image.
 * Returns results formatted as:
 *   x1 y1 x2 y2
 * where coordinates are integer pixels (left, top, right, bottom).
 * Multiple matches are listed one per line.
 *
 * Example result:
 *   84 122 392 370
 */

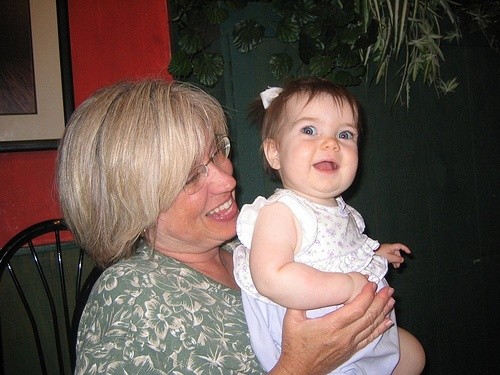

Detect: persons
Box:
230 77 426 375
52 77 397 375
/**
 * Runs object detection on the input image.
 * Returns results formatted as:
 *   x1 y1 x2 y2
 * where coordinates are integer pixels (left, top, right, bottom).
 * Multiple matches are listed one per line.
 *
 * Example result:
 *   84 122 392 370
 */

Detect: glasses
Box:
181 137 232 197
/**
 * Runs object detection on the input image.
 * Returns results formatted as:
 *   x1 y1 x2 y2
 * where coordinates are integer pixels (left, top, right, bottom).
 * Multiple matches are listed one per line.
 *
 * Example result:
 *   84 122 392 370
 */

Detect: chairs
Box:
0 217 108 375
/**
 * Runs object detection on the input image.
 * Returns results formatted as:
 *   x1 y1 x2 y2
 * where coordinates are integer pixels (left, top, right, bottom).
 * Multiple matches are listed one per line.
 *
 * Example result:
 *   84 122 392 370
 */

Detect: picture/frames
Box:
0 0 77 152
